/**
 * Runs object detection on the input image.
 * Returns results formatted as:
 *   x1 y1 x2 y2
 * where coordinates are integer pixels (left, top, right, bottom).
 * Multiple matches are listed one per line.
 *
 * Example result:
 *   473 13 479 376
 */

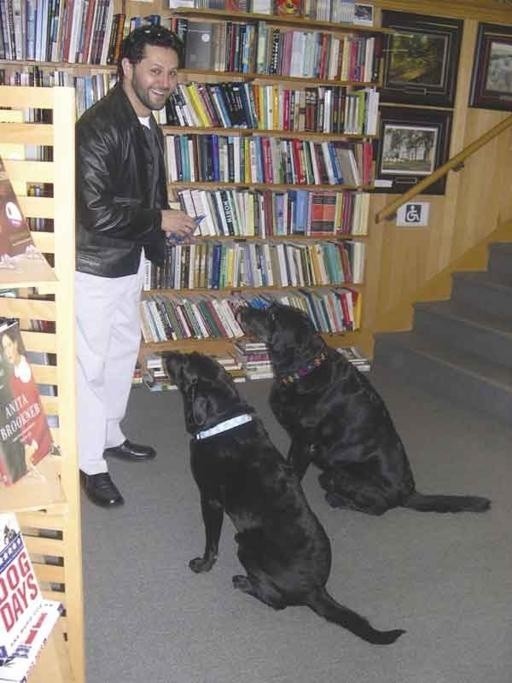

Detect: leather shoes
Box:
79 470 125 510
103 439 156 464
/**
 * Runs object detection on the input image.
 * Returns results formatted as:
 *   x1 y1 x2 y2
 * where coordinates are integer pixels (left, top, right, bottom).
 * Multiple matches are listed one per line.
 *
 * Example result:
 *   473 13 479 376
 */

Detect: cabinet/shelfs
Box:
0 0 383 377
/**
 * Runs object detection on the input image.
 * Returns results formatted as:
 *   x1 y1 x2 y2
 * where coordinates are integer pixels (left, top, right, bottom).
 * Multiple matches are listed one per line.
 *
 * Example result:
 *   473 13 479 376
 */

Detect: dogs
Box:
234 302 491 515
161 349 408 644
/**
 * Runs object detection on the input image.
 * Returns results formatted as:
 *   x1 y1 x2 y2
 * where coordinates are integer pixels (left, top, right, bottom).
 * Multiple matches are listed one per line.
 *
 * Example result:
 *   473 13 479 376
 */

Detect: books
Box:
137 134 372 292
140 285 372 344
0 512 46 661
0 597 63 683
338 346 371 372
1 0 387 83
2 66 379 136
2 323 55 488
134 341 276 392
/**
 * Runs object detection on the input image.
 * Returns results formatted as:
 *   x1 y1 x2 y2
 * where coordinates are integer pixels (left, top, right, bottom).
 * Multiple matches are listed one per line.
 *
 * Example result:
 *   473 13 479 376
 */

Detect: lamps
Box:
0 87 89 682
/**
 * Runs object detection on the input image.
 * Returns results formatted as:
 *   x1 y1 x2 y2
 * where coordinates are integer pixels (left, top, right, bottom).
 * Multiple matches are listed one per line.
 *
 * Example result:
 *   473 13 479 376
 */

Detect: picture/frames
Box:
376 8 464 109
468 22 512 113
362 105 454 196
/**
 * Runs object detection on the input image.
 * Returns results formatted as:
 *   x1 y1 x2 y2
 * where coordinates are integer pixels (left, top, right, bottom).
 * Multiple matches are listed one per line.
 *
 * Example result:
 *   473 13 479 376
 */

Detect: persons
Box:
73 21 202 508
